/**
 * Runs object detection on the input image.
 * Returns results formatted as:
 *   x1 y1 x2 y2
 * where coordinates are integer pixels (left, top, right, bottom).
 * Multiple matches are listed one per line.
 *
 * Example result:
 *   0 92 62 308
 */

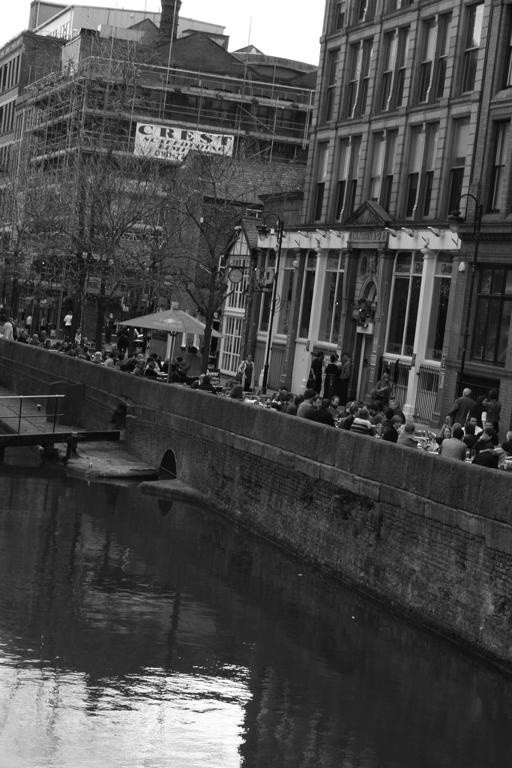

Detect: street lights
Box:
449 194 483 397
256 213 284 393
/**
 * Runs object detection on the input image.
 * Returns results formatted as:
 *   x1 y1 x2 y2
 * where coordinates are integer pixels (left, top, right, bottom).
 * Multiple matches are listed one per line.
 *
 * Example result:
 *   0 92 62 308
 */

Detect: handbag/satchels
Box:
371 388 379 399
235 372 242 382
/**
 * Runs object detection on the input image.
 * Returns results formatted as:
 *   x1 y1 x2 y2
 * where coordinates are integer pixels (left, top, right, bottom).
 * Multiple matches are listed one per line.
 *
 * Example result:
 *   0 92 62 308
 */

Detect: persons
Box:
1 311 146 377
271 351 512 471
144 346 255 400
